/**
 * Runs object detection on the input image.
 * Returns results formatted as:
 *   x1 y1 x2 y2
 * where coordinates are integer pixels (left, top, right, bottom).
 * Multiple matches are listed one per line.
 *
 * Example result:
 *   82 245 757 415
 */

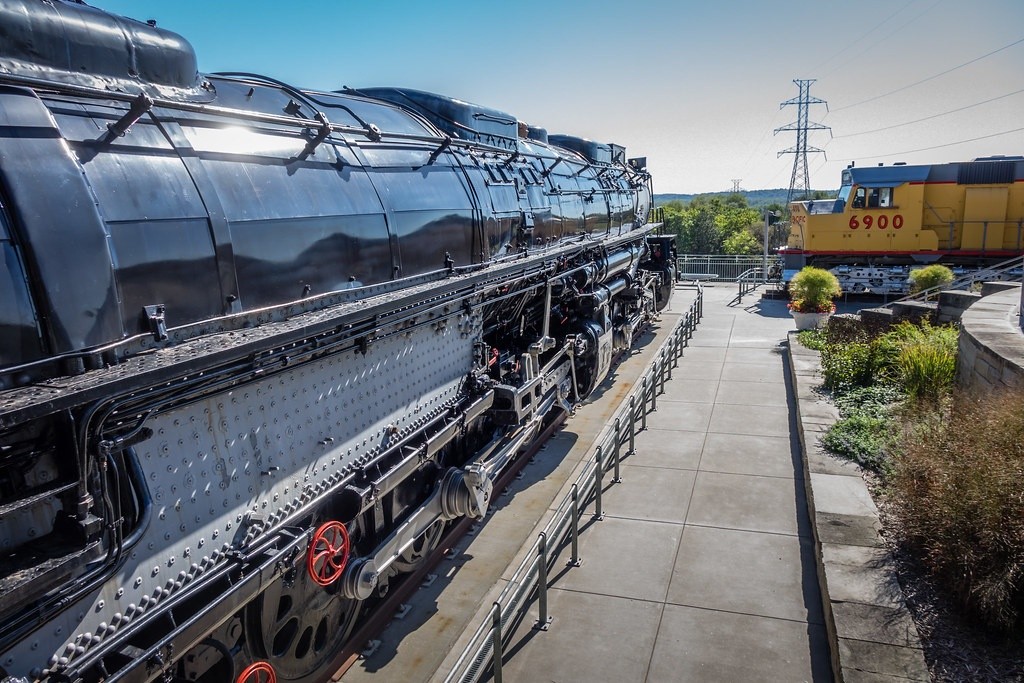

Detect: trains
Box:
767 155 1024 295
0 1 681 683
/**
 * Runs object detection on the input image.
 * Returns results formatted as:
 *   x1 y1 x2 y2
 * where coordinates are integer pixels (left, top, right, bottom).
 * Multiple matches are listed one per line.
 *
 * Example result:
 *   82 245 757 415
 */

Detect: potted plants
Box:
788 265 843 331
